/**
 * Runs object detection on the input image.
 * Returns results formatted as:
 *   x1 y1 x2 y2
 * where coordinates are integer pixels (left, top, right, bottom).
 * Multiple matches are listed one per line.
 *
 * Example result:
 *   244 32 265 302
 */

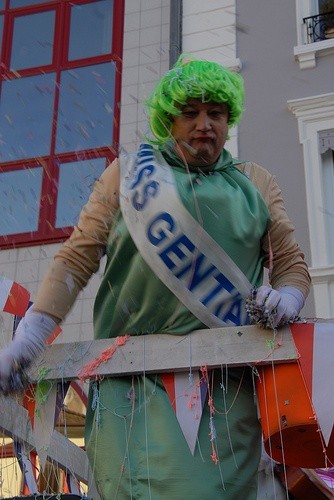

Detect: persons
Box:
0 60 312 500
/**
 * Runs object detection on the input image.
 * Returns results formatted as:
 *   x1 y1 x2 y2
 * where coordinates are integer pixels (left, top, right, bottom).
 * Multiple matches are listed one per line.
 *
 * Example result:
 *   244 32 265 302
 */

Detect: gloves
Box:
246 286 305 331
0 311 57 393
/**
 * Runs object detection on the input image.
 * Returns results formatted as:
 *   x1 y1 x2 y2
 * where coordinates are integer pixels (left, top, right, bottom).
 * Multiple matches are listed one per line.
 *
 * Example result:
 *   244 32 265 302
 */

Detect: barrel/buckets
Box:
255 321 334 469
